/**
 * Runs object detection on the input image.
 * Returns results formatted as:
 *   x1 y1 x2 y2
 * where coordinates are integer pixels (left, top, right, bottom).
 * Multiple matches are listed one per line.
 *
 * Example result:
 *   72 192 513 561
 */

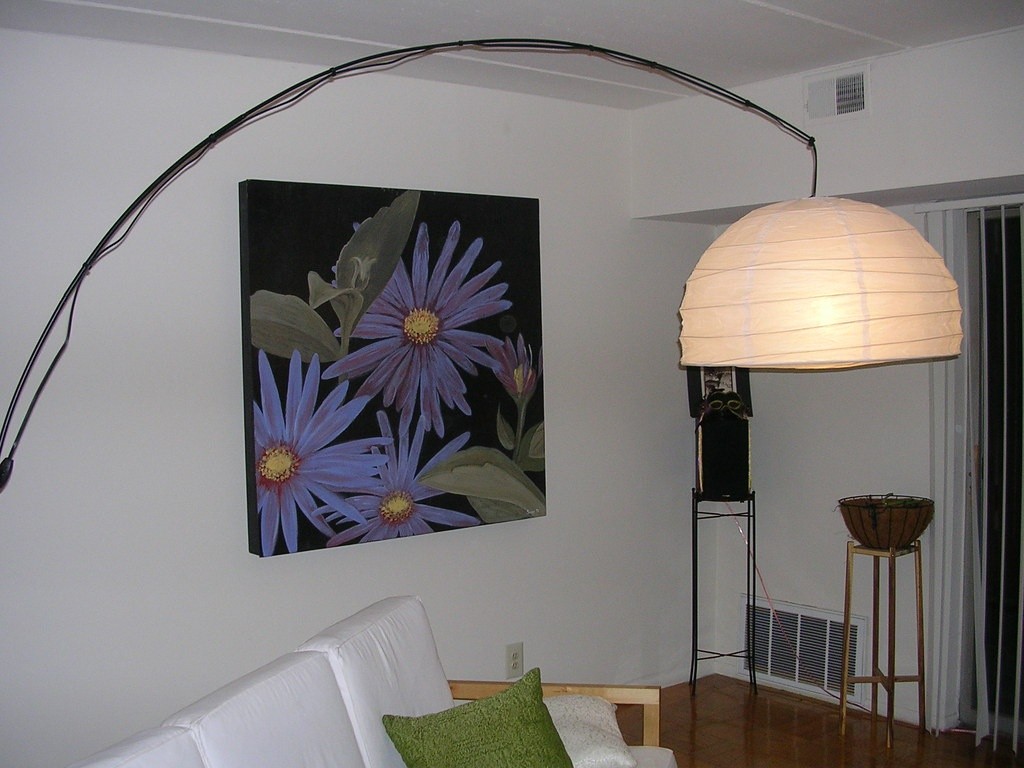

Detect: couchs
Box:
65 593 677 768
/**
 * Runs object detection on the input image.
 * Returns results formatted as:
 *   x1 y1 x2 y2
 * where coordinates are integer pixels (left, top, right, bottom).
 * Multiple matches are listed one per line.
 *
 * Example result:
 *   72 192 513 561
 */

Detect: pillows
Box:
383 666 574 768
542 694 638 768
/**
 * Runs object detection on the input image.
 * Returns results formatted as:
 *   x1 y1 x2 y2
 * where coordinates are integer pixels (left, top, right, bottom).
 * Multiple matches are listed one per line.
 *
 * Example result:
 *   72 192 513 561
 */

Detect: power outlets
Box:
506 643 522 680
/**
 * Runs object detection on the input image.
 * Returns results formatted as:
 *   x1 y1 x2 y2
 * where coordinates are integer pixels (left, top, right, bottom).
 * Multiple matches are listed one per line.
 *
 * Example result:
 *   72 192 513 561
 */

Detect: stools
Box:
841 541 928 750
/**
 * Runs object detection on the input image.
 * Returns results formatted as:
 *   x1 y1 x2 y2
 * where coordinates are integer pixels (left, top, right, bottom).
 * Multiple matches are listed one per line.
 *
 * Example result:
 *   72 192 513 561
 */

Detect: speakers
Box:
696 419 750 498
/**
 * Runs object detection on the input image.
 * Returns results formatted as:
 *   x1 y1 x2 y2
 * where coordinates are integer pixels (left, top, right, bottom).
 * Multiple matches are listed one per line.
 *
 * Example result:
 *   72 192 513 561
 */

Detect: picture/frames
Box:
686 366 753 420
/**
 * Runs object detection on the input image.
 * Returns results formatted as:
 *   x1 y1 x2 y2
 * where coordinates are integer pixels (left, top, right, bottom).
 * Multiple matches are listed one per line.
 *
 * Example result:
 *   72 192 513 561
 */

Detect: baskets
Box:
837 493 936 551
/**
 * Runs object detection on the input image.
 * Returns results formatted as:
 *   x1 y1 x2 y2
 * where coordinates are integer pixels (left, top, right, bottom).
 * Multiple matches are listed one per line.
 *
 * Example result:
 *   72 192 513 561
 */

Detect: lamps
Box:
0 36 962 493
